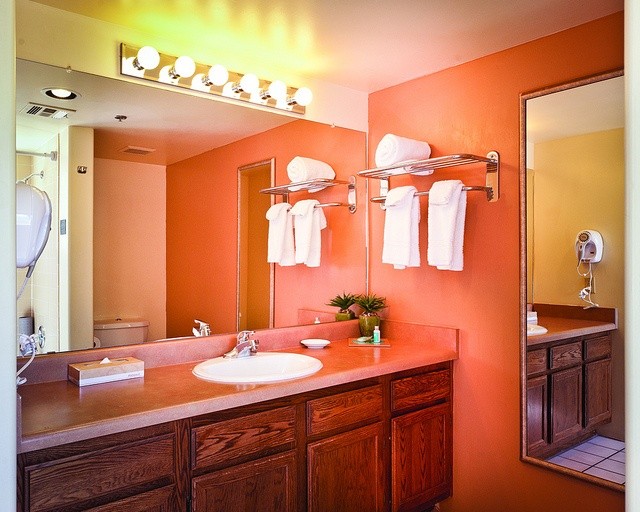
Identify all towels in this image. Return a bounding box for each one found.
[375,133,436,176]
[265,203,295,268]
[290,199,326,269]
[286,157,335,193]
[427,180,468,271]
[381,186,422,271]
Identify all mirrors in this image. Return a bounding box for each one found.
[518,68,625,491]
[17,59,367,357]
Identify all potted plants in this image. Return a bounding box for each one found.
[326,290,363,321]
[355,292,389,340]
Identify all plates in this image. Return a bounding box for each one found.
[301,339,331,349]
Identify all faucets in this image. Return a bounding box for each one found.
[235,326,261,353]
[192,319,210,337]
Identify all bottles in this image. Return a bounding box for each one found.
[314,318,320,323]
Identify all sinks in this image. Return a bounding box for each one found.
[193,350,323,385]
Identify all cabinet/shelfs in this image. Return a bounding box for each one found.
[306,374,386,510]
[583,330,613,430]
[523,343,548,454]
[391,359,454,511]
[551,339,582,442]
[188,392,307,511]
[34,418,188,510]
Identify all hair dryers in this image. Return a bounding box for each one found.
[578,231,590,265]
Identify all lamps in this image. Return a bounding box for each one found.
[268,81,286,101]
[170,57,196,79]
[208,65,228,88]
[294,88,312,107]
[239,72,259,96]
[132,45,161,72]
